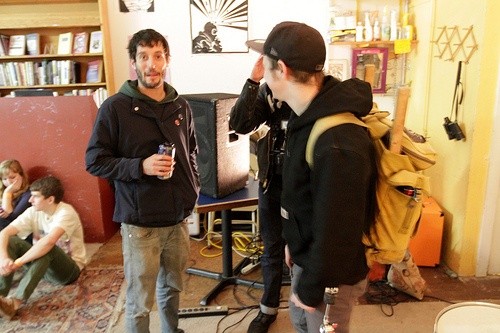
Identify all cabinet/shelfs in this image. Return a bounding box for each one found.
[408,196,444,267]
[0,0,114,110]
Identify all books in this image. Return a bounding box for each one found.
[0,31,103,97]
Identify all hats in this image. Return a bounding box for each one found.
[246,21,327,71]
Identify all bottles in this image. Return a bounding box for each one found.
[355,22,363,41]
[355,61,365,80]
[156,143,176,179]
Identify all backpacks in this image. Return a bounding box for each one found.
[305,103,437,264]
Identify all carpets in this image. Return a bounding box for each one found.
[0,263,128,333]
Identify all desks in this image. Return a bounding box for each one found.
[183,174,264,306]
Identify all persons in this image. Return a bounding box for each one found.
[245,21,376,333]
[228,56,292,333]
[0,160,32,231]
[0,175,86,320]
[85,30,201,333]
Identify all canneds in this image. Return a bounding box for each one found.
[157,142,175,180]
[402,189,414,197]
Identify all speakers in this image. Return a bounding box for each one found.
[179,92,249,199]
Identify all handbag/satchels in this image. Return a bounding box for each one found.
[387,251,428,300]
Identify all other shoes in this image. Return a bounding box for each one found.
[247,311,277,333]
[0,295,18,320]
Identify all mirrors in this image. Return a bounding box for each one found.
[352,47,388,94]
